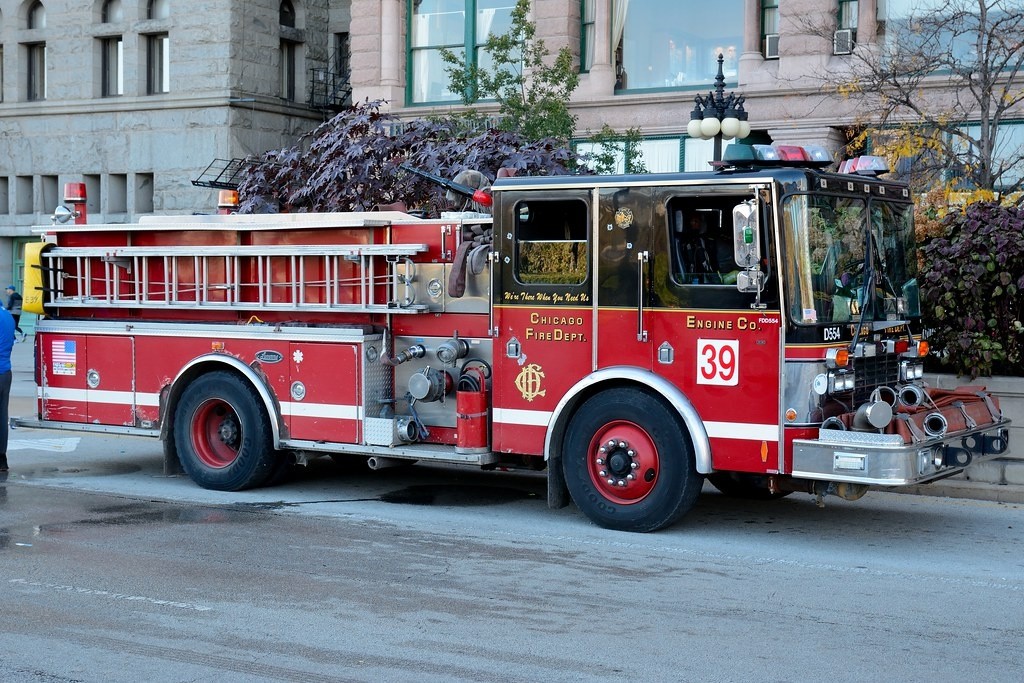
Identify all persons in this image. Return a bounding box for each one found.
[0,300,16,470]
[5,285,27,343]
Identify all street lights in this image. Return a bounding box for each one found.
[687,52,752,172]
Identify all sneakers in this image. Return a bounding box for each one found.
[14,339,19,344]
[21,332,27,342]
[0,463,9,471]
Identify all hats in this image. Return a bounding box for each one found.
[5,285,16,291]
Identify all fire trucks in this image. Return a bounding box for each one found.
[9,142,1015,534]
